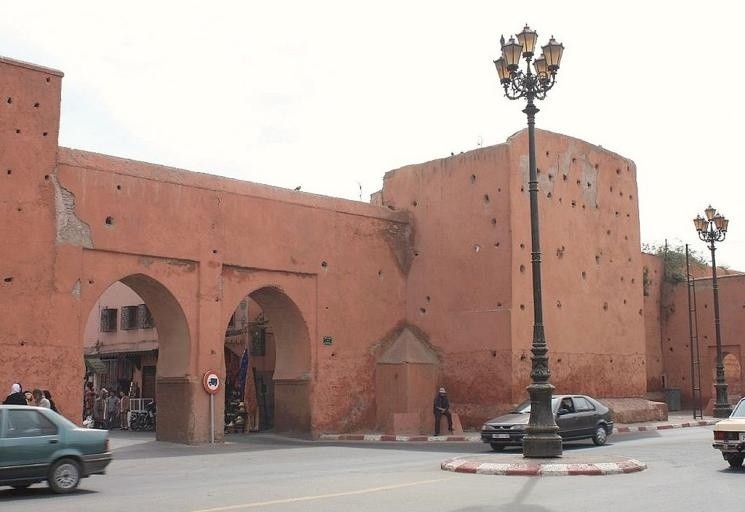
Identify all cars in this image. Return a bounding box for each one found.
[0,405,113,494]
[480,395,614,449]
[712,394,745,468]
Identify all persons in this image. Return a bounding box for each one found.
[434,388,456,436]
[2,382,59,413]
[82,385,130,431]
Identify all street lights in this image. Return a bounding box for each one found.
[694,202,730,419]
[495,20,565,458]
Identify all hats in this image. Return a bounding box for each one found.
[439,388,446,394]
